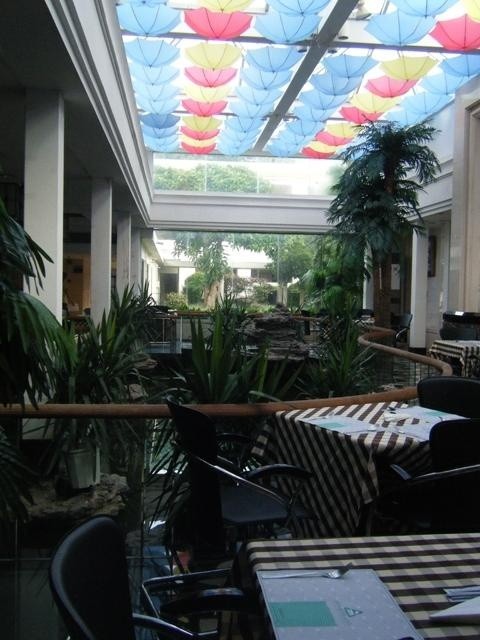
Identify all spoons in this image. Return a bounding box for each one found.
[343,423,382,435]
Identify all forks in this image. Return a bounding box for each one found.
[307,411,336,420]
[261,561,353,580]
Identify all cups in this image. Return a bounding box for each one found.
[407,397,420,410]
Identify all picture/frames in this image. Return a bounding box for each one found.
[428,236,436,278]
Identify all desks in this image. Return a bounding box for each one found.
[427,339,480,376]
[229,533,480,639]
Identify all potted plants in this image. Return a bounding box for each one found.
[44,280,169,493]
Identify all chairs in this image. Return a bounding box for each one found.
[389,312,412,346]
[45,514,246,640]
